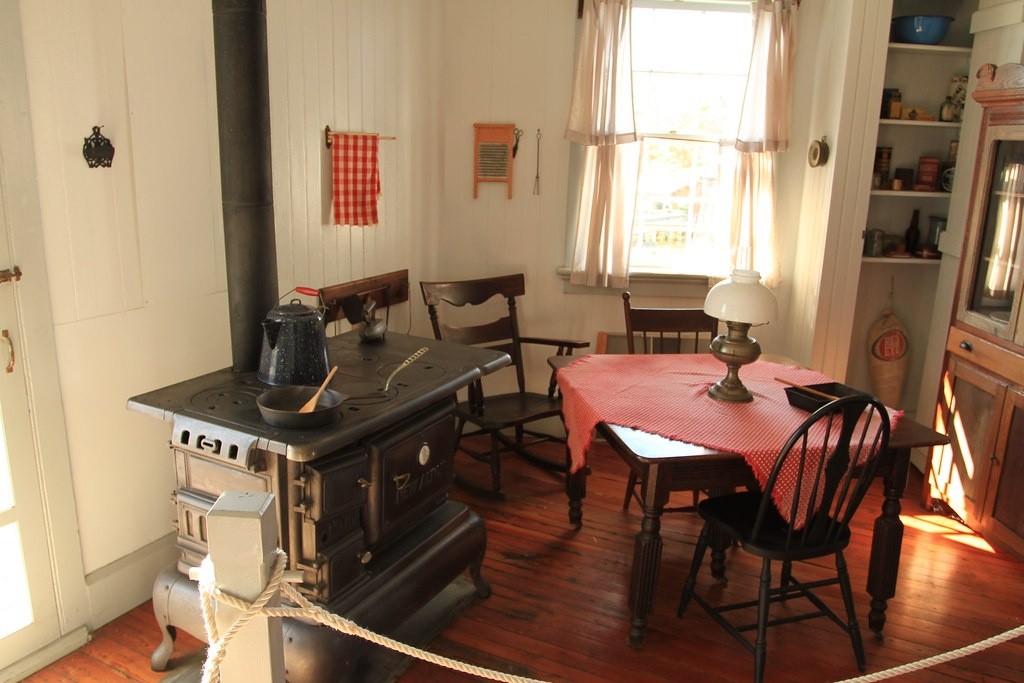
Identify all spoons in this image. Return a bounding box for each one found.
[298,366,338,412]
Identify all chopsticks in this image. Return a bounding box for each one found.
[774,376,847,400]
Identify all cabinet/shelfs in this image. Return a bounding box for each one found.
[923,89,1024,558]
[858,44,972,264]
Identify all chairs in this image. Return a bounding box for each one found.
[675,394,891,683]
[620,292,729,513]
[418,272,591,501]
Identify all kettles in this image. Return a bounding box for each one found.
[257,286,330,389]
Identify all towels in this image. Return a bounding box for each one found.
[332,132,383,228]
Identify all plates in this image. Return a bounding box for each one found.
[989,311,1011,323]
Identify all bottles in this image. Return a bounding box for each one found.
[939,96,956,122]
[904,208,921,255]
[938,140,958,192]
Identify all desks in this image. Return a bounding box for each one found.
[554,350,950,650]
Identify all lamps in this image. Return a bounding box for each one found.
[703,269,778,403]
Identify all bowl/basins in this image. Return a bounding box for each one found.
[255,386,350,430]
[892,15,955,45]
[783,382,867,415]
